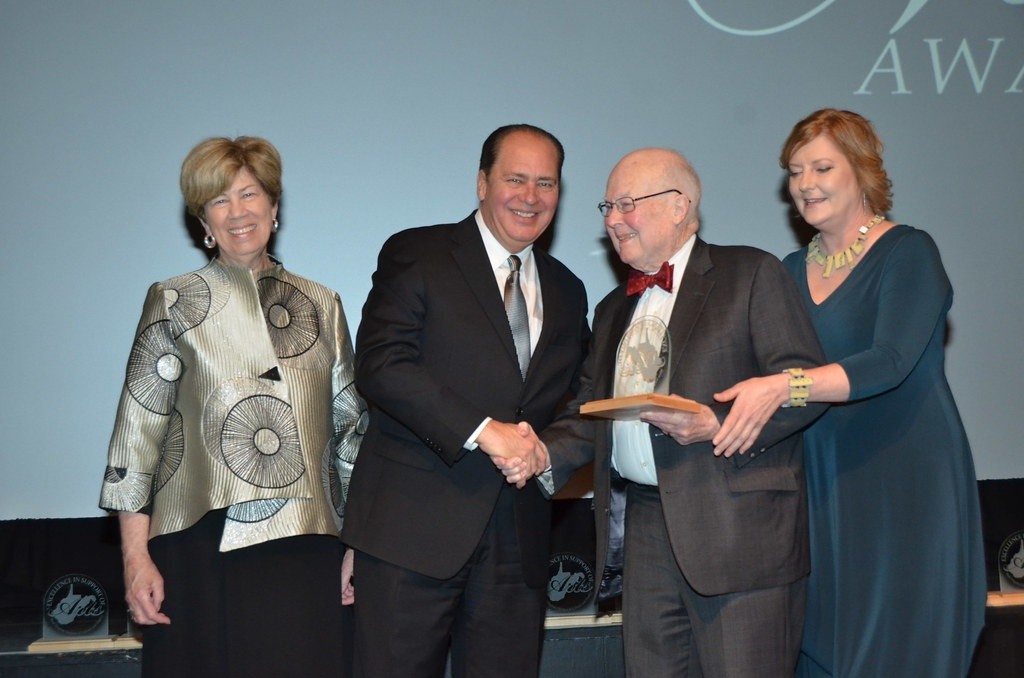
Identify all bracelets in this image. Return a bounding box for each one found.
[784,366,813,409]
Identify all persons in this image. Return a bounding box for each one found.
[98,136,368,678]
[343,125,591,678]
[489,107,987,678]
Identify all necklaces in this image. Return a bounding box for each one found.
[802,215,889,276]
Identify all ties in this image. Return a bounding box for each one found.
[504,255,531,382]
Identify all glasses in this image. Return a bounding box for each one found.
[598,190,691,217]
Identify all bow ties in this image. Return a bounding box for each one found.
[626,262,674,297]
